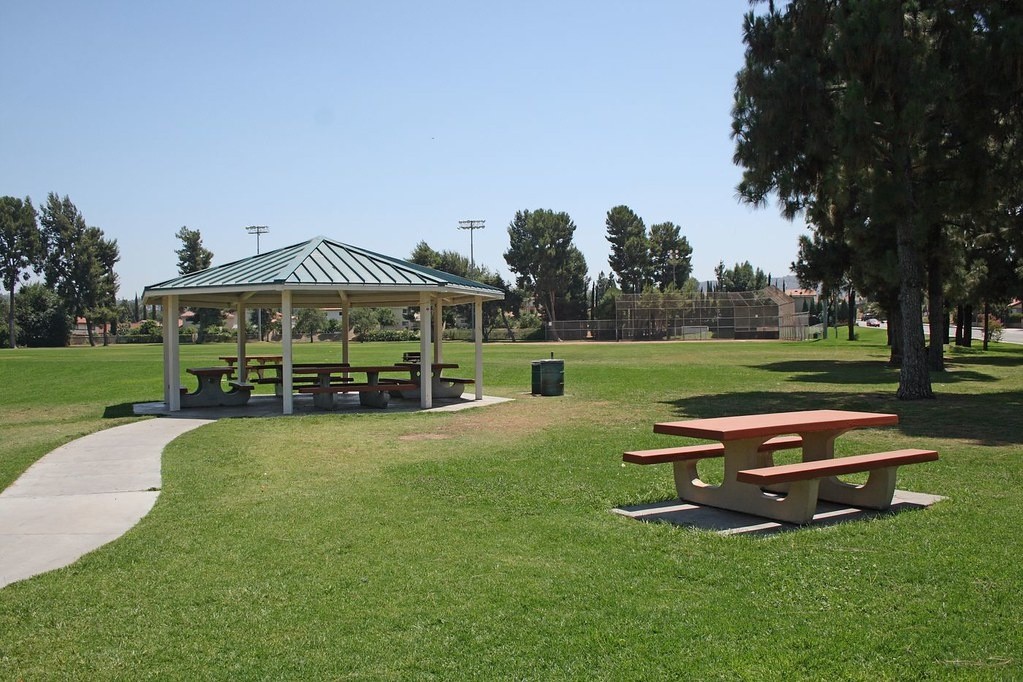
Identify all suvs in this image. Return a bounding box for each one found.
[861,313,872,321]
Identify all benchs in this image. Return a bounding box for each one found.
[622,437,802,504]
[737,449,940,524]
[173,375,476,409]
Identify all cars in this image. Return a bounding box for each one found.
[866,318,881,327]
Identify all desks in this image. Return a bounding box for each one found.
[293,366,410,409]
[184,368,234,406]
[249,361,349,398]
[219,356,282,380]
[653,409,900,523]
[395,359,459,399]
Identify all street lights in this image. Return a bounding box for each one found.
[669,249,679,340]
[246,225,270,341]
[457,219,486,341]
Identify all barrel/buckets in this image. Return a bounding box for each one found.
[532,360,541,394]
[541,358,565,396]
